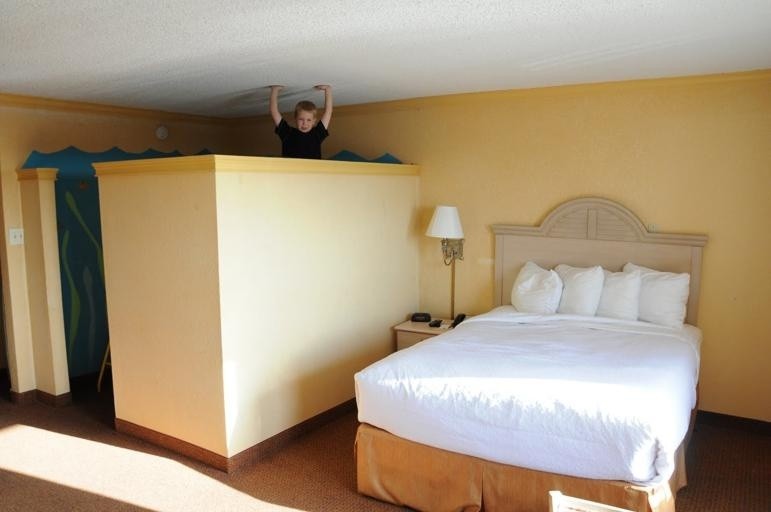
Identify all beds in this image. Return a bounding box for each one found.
[353,197,708,512]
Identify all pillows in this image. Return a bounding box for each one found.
[596,269,640,323]
[510,261,563,315]
[623,262,690,330]
[554,263,605,317]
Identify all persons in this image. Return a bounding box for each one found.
[269,84,333,159]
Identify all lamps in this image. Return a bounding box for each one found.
[424,205,464,326]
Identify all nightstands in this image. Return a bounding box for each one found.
[393,318,454,351]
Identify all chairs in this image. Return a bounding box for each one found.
[549,489,633,512]
